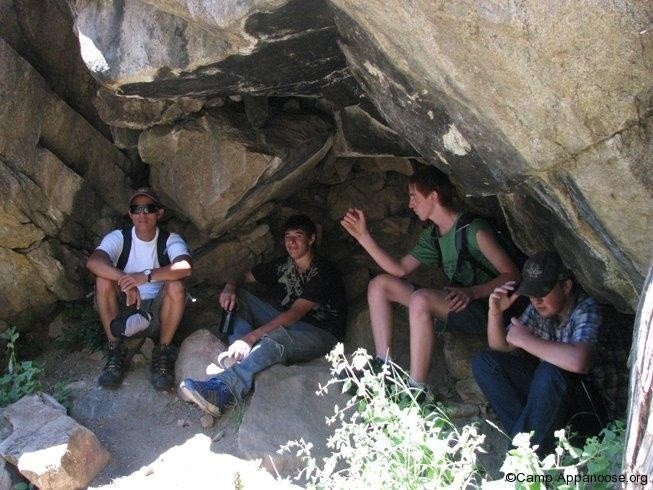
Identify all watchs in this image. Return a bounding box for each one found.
[142,267,152,282]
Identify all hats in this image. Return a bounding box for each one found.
[515,250,567,298]
[128,188,160,205]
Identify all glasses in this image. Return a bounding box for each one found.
[130,205,159,214]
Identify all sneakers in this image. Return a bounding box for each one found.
[217,351,240,370]
[151,343,177,391]
[180,377,231,418]
[341,359,427,405]
[99,340,130,388]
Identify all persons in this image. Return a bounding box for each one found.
[338,164,533,408]
[84,186,195,391]
[178,213,348,418]
[470,247,630,470]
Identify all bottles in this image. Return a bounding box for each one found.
[218,302,238,335]
